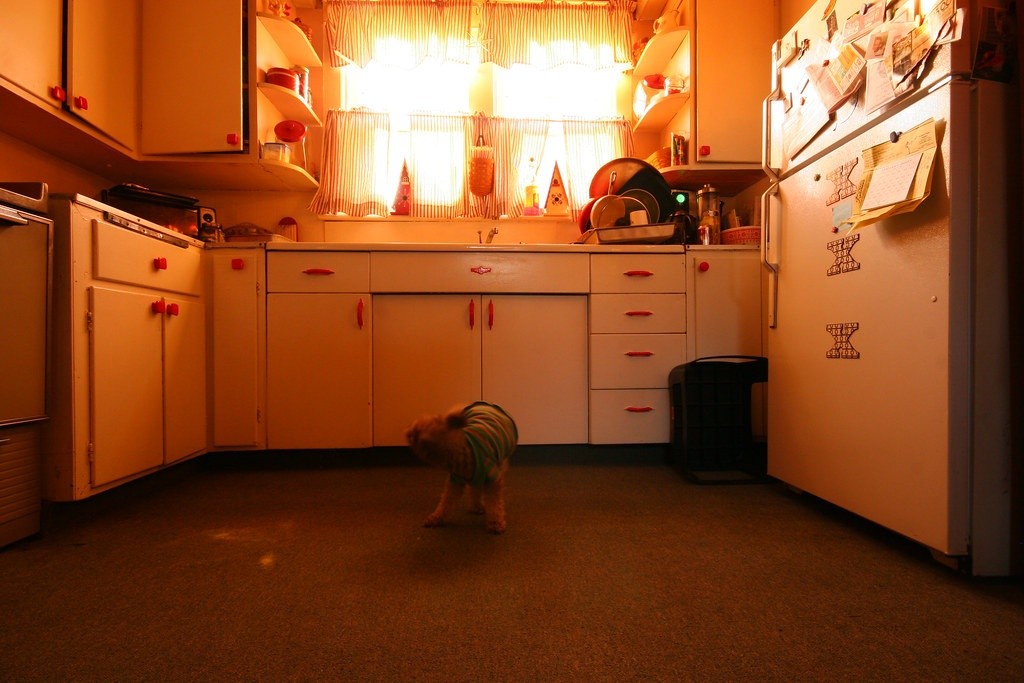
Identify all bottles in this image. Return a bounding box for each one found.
[696,183,721,245]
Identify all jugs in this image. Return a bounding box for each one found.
[652,9,680,35]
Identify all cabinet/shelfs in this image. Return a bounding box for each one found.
[0,0,783,547]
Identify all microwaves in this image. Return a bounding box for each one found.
[100,185,216,245]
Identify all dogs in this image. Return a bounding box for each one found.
[405,401,519,536]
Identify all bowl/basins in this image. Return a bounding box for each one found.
[722,226,763,247]
[577,189,659,235]
